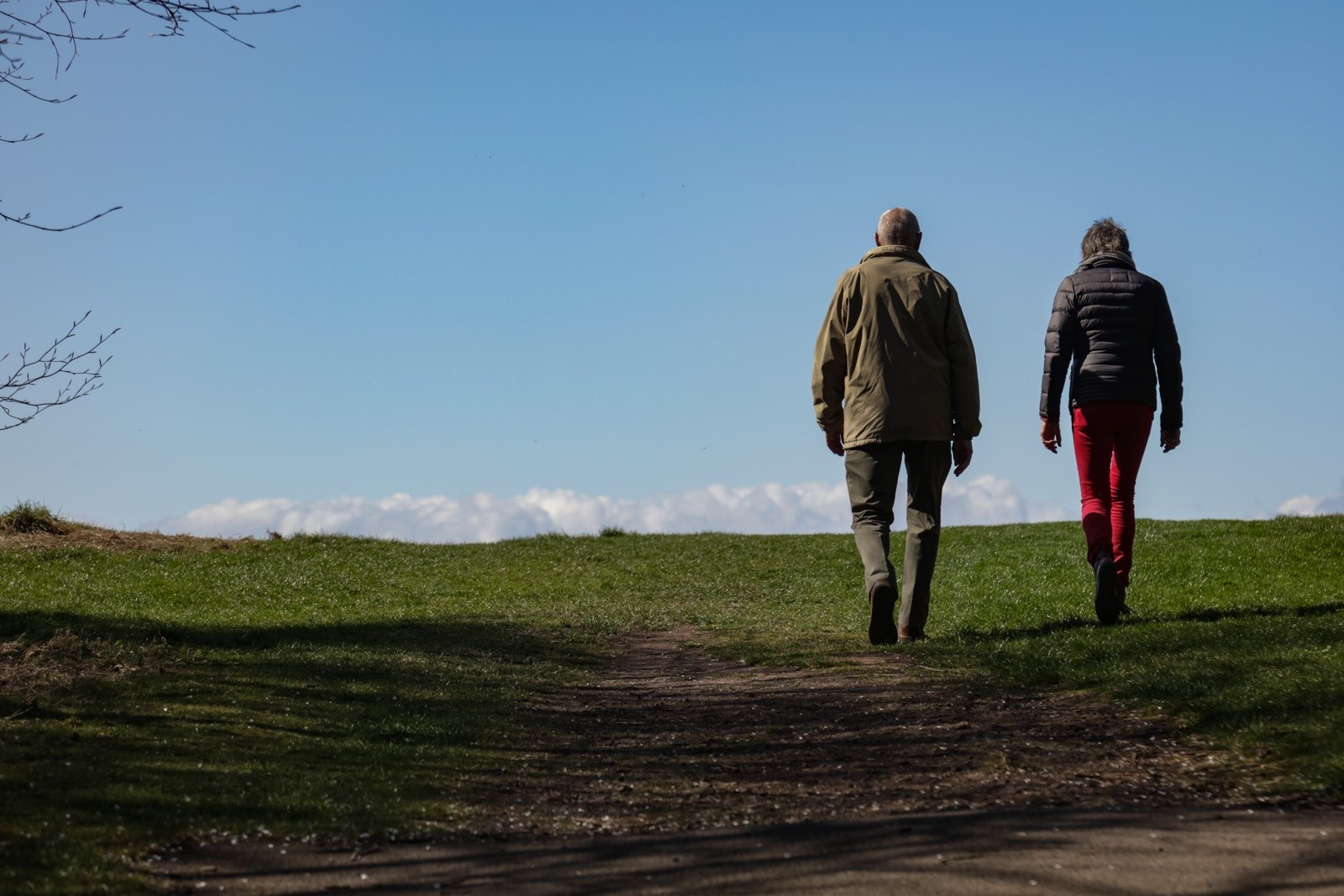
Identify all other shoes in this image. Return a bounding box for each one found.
[1095,558,1120,626]
[899,623,924,643]
[869,580,898,644]
[1119,603,1131,615]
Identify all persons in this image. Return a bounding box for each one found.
[1039,217,1184,626]
[812,207,982,645]
